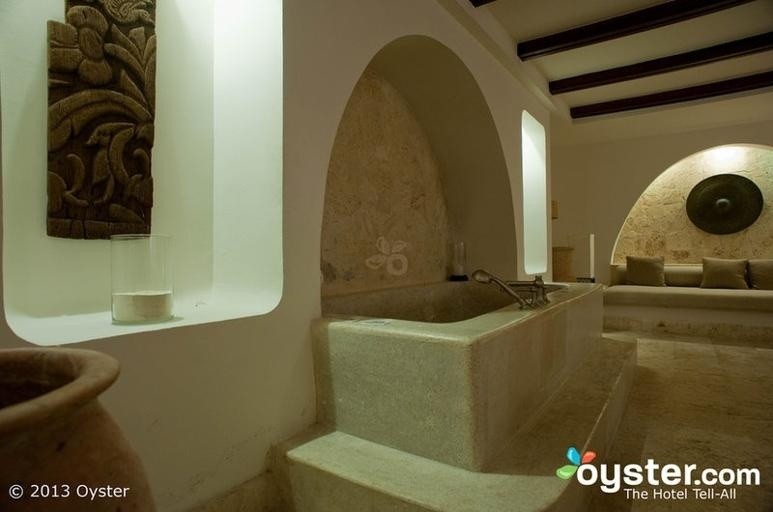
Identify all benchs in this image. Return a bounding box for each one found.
[603,265,773,340]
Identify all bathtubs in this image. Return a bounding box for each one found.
[311,276,604,471]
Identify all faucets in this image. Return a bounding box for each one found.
[472,267,534,313]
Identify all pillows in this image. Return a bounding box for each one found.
[748,259,773,289]
[699,257,749,289]
[626,255,666,287]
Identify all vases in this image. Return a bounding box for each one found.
[0,346,156,512]
